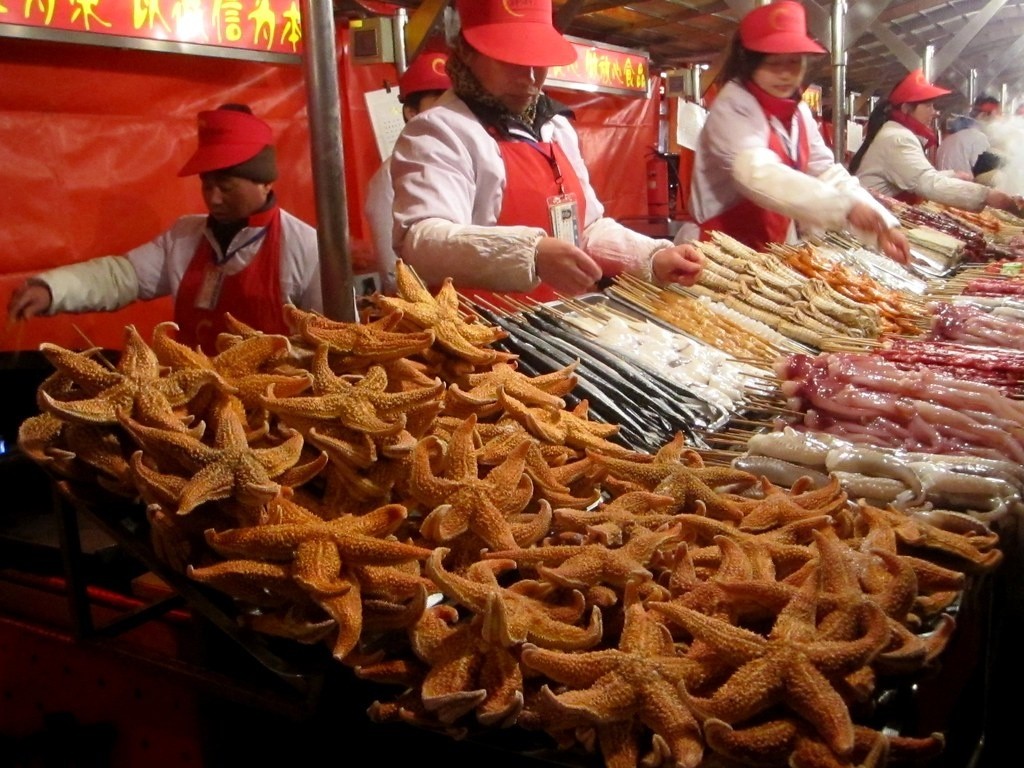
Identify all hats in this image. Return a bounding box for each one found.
[973,102,997,110]
[397,53,451,92]
[458,0,577,68]
[890,69,951,105]
[179,109,275,178]
[736,2,825,53]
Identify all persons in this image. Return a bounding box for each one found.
[562,210,571,218]
[363,52,1024,294]
[391,0,708,296]
[5,102,325,356]
[674,0,912,263]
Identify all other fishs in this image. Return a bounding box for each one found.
[472,192,1024,535]
